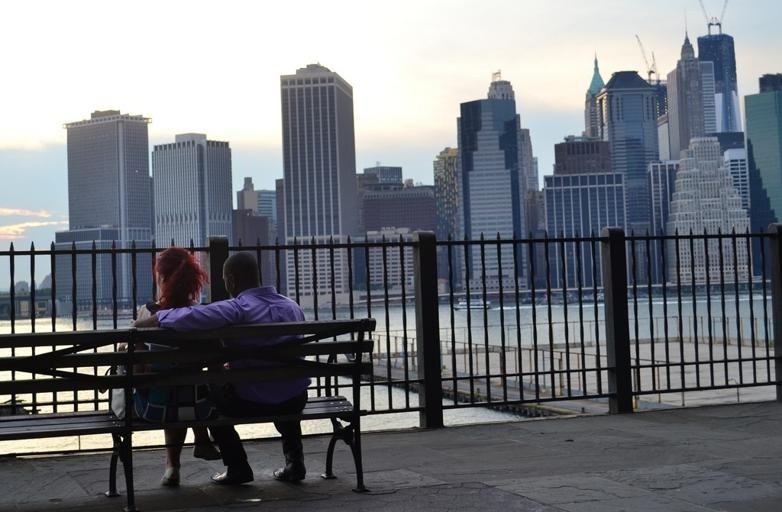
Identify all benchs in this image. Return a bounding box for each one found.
[0,318,377,512]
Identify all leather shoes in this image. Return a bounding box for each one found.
[210,470,255,485]
[273,463,306,481]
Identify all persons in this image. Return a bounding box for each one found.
[120,251,306,485]
[105,247,224,486]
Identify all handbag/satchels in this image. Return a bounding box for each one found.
[98,345,146,422]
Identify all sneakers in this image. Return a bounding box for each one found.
[193,445,223,461]
[161,467,180,486]
